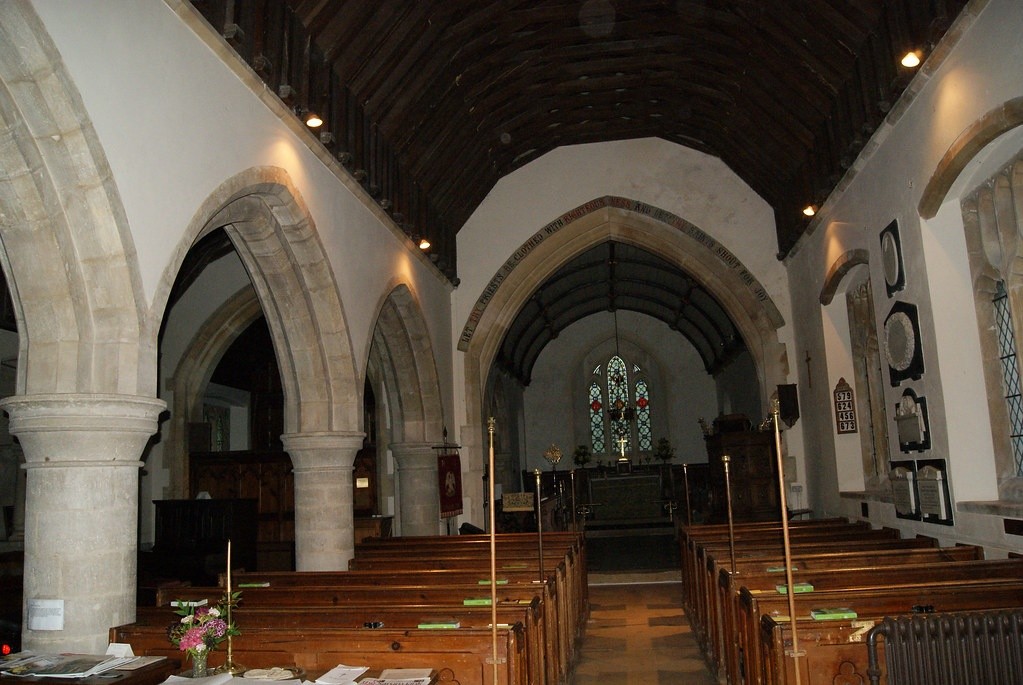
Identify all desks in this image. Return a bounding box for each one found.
[0,653,173,685]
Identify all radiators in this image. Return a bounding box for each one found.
[867,612,1023,685]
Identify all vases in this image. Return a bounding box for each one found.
[191,654,207,679]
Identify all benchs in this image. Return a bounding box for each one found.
[103,531,587,685]
[675,518,1023,683]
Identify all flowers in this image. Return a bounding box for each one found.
[171,588,244,660]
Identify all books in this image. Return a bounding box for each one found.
[418,621,460,628]
[464,596,499,605]
[171,598,208,606]
[157,673,301,685]
[0,653,167,678]
[479,578,508,584]
[315,663,370,685]
[489,623,512,626]
[238,581,270,587]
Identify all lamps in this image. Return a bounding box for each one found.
[417,238,430,251]
[802,203,818,216]
[302,111,323,127]
[901,49,924,68]
